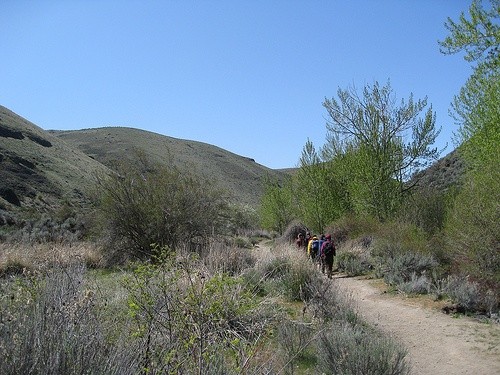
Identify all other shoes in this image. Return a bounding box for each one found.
[327,274,332,280]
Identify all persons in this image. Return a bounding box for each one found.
[322,233,336,279]
[295,231,326,273]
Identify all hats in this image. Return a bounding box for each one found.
[326,234,331,238]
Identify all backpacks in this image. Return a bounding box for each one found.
[325,241,333,256]
